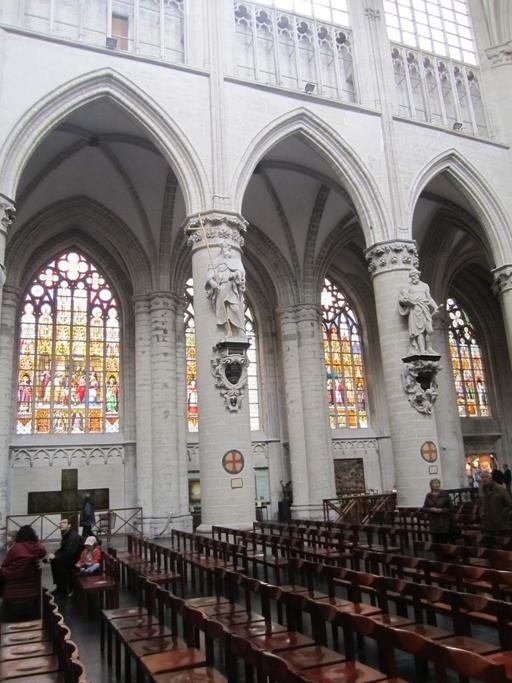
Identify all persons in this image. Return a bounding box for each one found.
[478,471,511,537]
[1,525,47,596]
[205,238,247,336]
[279,479,291,501]
[49,518,85,595]
[491,464,503,483]
[326,376,366,411]
[454,375,487,408]
[81,491,102,544]
[16,364,120,434]
[423,478,456,560]
[75,535,101,574]
[186,379,198,413]
[397,265,440,354]
[502,464,511,490]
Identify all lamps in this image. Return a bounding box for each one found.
[305,84,314,94]
[453,122,462,130]
[106,37,117,49]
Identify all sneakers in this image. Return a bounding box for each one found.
[80,567,90,575]
[51,588,77,596]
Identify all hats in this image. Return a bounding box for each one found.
[83,535,97,546]
